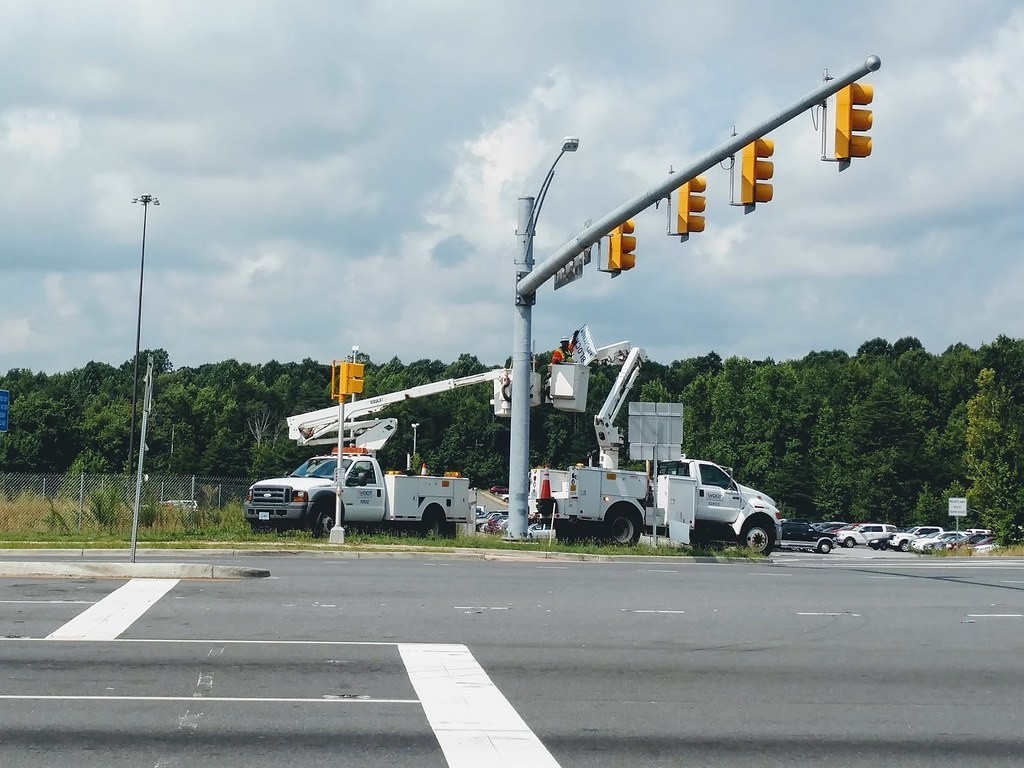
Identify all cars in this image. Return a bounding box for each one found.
[489,486,509,496]
[809,522,1002,554]
[472,493,557,543]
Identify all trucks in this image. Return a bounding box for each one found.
[242,362,513,540]
[525,322,784,560]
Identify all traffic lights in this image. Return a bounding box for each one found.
[740,138,775,203]
[677,175,707,233]
[337,359,365,397]
[607,219,637,270]
[833,81,874,161]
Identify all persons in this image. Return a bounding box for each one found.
[551,329,579,365]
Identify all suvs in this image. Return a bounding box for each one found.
[166,500,199,512]
[778,520,838,554]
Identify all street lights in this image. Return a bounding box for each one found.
[123,191,162,498]
[501,135,584,539]
[421,463,427,477]
[412,423,420,455]
